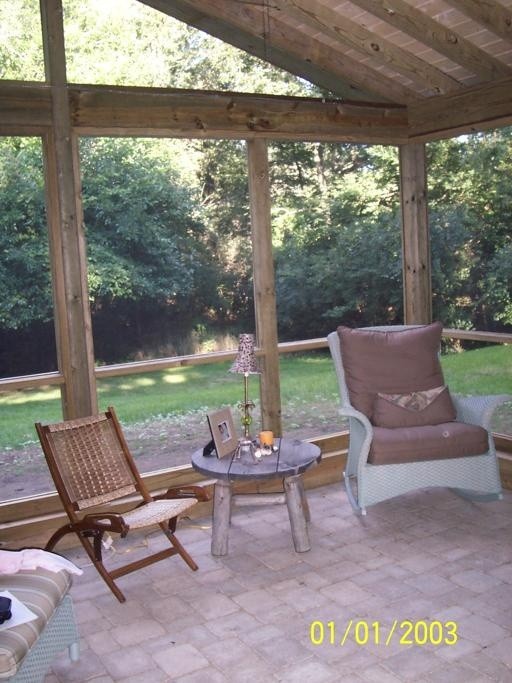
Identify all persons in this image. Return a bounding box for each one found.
[218,425,230,442]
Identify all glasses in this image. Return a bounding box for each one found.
[227,333,263,439]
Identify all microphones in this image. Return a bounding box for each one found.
[0,548,79,683]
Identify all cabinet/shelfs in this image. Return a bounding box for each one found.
[259,430,273,457]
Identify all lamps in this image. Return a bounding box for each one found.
[35,405,211,602]
[326,321,504,517]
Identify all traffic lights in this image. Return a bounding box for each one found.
[338,320,445,421]
[371,384,456,428]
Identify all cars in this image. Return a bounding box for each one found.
[235,437,247,459]
[240,442,258,466]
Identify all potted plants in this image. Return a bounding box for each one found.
[202,407,238,459]
